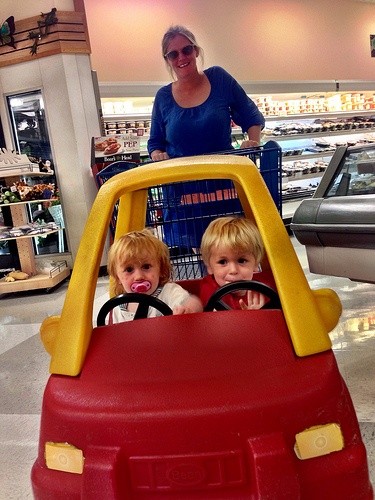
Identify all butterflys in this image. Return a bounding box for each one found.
[0,16,16,49]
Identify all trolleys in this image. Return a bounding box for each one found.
[92,141,284,355]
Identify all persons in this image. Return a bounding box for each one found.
[107,228,203,326]
[200,216,275,312]
[146,25,266,277]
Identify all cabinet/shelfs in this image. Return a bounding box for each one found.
[0,148,70,293]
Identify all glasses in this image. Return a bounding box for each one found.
[164,45,196,61]
